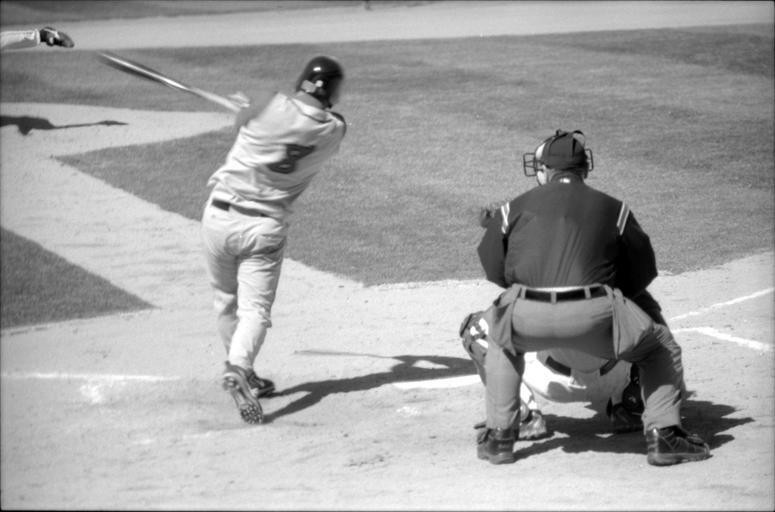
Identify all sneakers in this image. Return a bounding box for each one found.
[42,27,75,48]
[222,363,274,425]
[610,402,643,435]
[645,426,711,465]
[476,407,549,464]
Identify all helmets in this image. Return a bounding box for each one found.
[294,56,345,109]
[522,133,594,186]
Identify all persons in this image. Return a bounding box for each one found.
[478,129,710,465]
[460,289,667,439]
[201,56,346,425]
[0,27,74,50]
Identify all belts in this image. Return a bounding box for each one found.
[547,355,619,377]
[517,286,609,304]
[212,200,266,219]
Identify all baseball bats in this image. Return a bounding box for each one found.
[93,51,246,116]
